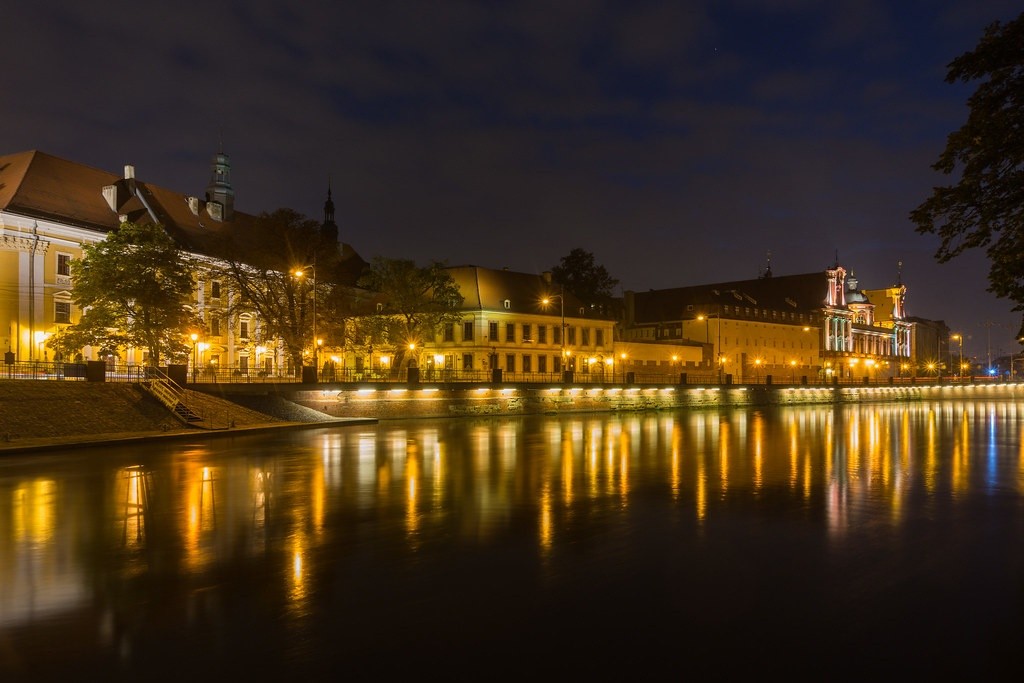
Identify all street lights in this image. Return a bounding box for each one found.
[929,364,934,386]
[952,335,962,385]
[804,327,825,386]
[409,343,415,359]
[698,313,721,384]
[673,355,678,382]
[191,333,198,384]
[904,364,908,377]
[963,364,968,377]
[543,295,566,381]
[722,359,726,383]
[565,351,571,371]
[295,264,316,366]
[1004,325,1018,380]
[977,322,1000,376]
[875,364,878,386]
[755,359,762,383]
[791,360,795,385]
[492,347,496,381]
[318,339,323,381]
[621,354,626,384]
